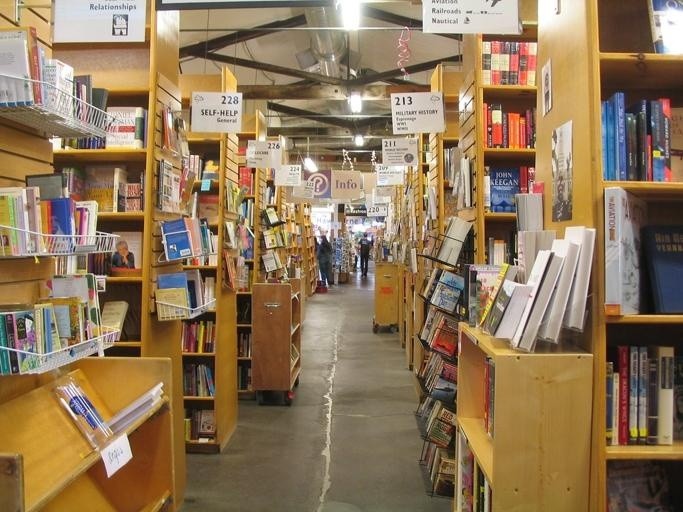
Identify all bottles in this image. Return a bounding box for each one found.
[53,370,115,451]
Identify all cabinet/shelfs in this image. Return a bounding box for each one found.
[1,1,187,511]
[236,110,319,402]
[373,0,681,512]
[330,236,354,276]
[176,70,238,455]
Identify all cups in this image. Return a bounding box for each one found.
[294,268,300,279]
[1,452,26,512]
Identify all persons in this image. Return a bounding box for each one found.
[352,230,383,278]
[314,236,334,284]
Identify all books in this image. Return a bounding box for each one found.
[388,0,683,512]
[0,26,320,440]
